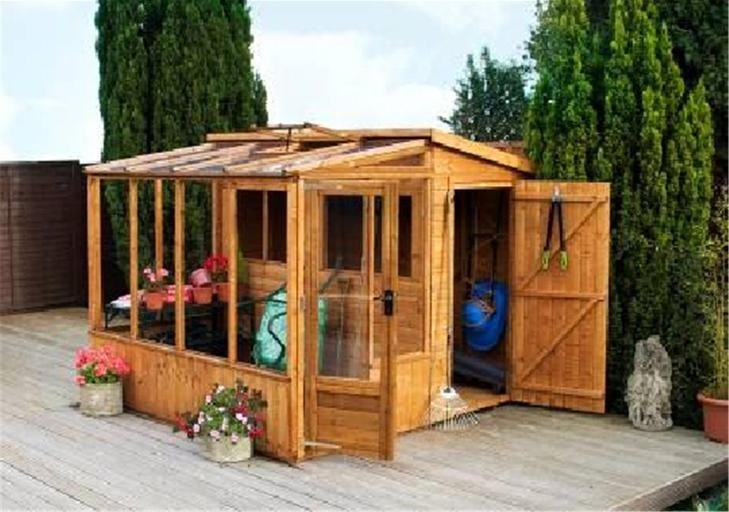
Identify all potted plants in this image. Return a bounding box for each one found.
[689,185,729,443]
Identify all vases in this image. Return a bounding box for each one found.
[191,268,212,286]
[81,383,123,417]
[191,285,213,305]
[146,292,163,309]
[208,436,252,463]
[216,282,229,302]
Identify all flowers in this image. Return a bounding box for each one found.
[75,343,131,385]
[140,267,169,291]
[174,380,267,443]
[204,253,228,282]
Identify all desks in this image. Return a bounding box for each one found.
[105,292,255,356]
